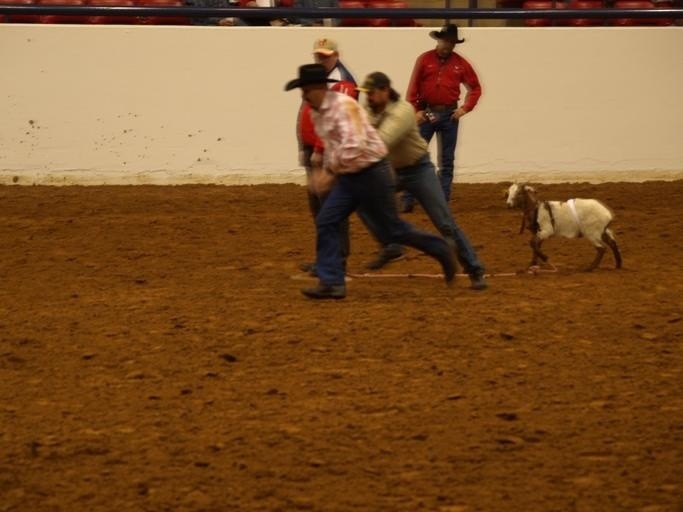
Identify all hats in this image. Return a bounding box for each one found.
[314,36,339,57]
[429,23,465,44]
[283,63,340,92]
[353,71,389,92]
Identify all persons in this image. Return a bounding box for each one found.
[353,71,487,290]
[1,1,682,26]
[399,24,481,214]
[296,37,360,279]
[284,63,459,299]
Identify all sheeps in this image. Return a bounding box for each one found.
[502,183,622,272]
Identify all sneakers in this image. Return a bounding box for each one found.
[441,244,457,281]
[300,262,317,274]
[367,252,404,270]
[301,284,345,298]
[468,268,486,289]
